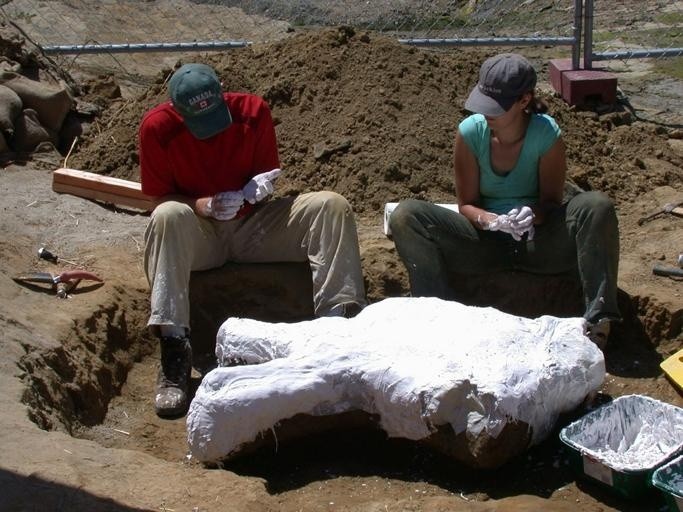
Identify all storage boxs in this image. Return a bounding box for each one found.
[558,393,683,499]
[651,455,683,512]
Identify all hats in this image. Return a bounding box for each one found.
[169,62,232,141]
[465,54,536,115]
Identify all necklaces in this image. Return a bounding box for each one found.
[491,128,527,147]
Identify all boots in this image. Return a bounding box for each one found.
[154,335,195,416]
[330,301,365,318]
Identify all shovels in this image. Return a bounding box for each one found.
[11,270,103,282]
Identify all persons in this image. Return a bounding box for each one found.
[137,62,369,418]
[388,51,629,366]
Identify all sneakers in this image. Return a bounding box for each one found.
[585,313,623,348]
